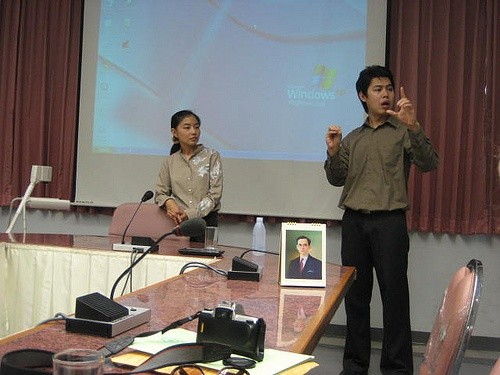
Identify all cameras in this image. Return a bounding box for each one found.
[197,300,267,362]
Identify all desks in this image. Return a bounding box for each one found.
[0,231,357,375]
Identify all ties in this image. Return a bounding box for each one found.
[299,258,305,272]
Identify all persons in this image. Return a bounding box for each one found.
[154,110,223,243]
[324,64,439,375]
[288,235,322,279]
[284,295,321,333]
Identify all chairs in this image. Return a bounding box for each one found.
[110,203,190,240]
[418,258,484,375]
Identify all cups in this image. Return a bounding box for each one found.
[205,226,219,251]
[52,349,105,375]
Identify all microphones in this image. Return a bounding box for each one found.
[121,190,154,244]
[110,217,206,300]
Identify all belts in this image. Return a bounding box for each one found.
[345,207,405,215]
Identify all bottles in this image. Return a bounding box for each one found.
[252,217,266,256]
